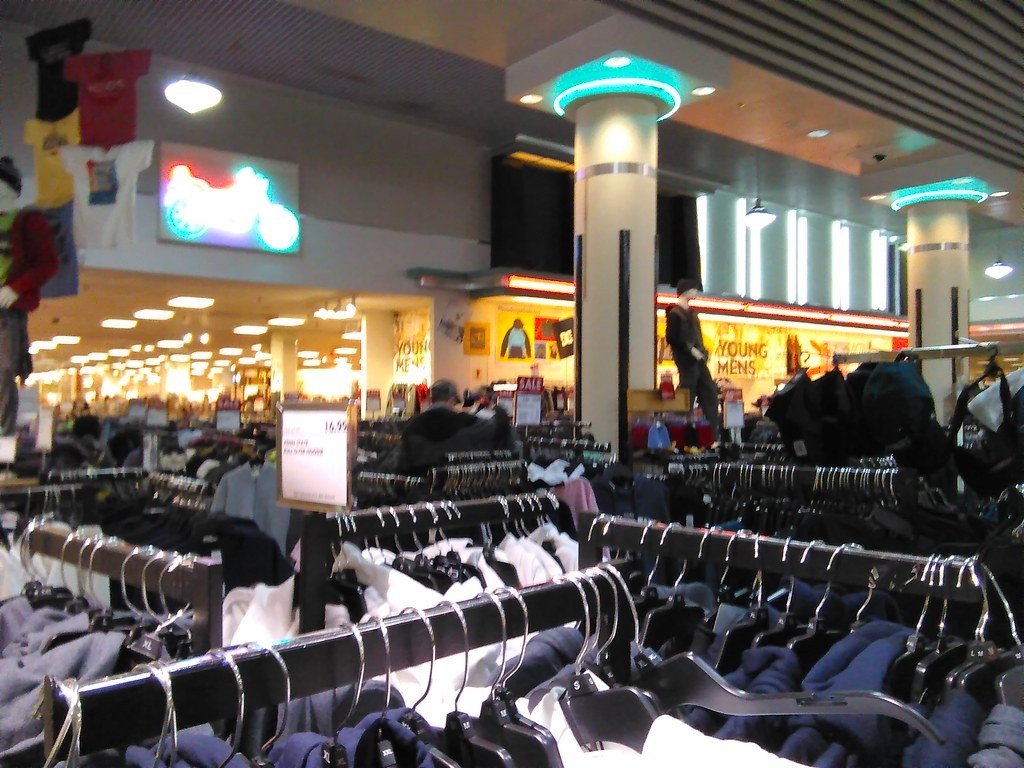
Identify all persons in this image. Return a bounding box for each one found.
[399,379,481,475]
[666,279,721,452]
[0,154,59,437]
[73,414,142,464]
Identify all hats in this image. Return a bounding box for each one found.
[0,155,21,192]
[778,356,945,466]
[431,382,461,405]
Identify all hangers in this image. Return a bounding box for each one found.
[1,339,1024,768]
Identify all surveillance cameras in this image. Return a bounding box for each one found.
[873,154,885,162]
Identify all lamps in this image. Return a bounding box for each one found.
[985,224,1013,280]
[164,56,224,115]
[742,155,778,229]
